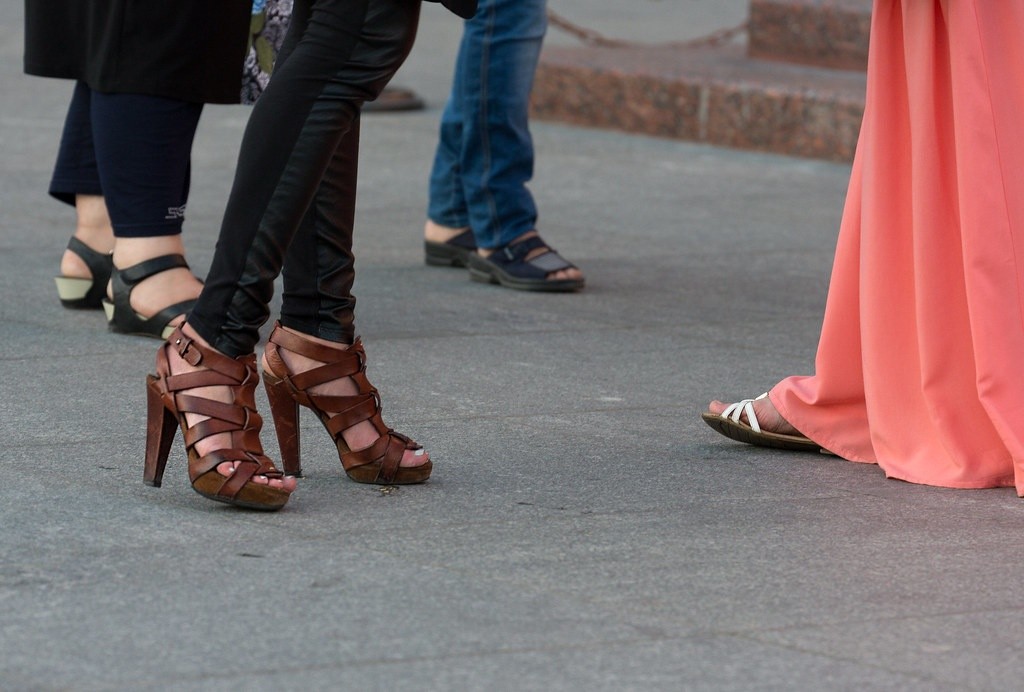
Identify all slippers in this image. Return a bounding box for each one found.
[422,231,475,266]
[467,228,585,292]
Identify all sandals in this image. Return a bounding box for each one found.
[58,235,207,342]
[702,391,846,450]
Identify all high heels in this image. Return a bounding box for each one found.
[261,321,432,486]
[142,317,292,511]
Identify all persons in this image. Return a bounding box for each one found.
[140,0,433,513]
[424,0,585,292]
[702,0,1023,498]
[24,0,255,339]
